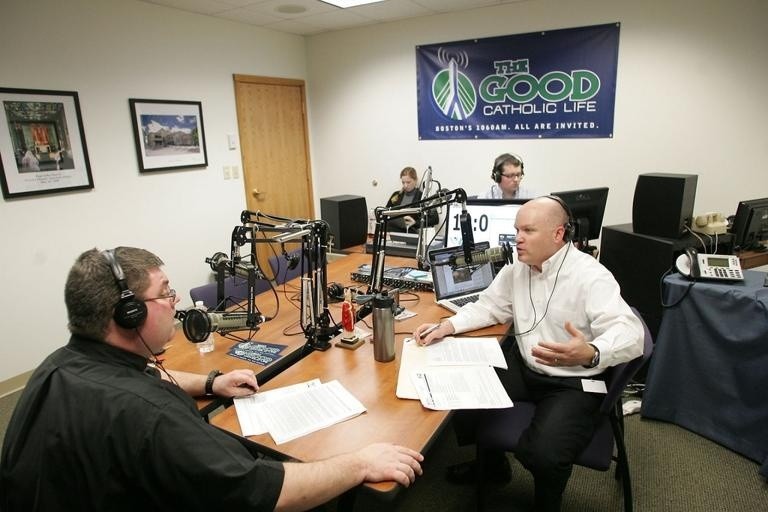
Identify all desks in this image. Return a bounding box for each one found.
[155,253,419,416]
[658,267,767,473]
[308,232,598,260]
[728,236,767,276]
[208,289,515,506]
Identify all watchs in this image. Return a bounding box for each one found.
[582,343,600,369]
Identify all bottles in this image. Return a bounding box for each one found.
[371,295,394,361]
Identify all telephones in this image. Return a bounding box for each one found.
[691,211,728,235]
[676,247,744,281]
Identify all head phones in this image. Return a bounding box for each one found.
[101,249,147,329]
[280,220,319,270]
[542,194,576,242]
[491,153,524,183]
[328,282,365,303]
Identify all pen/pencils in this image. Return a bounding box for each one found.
[407,323,440,343]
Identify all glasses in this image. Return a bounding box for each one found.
[142,289,176,302]
[501,172,522,179]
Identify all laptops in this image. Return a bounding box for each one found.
[429,241,496,314]
[383,217,407,233]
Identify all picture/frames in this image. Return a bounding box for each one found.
[127,97,208,174]
[0,87,95,199]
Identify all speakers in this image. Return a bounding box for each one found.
[320,194,368,250]
[633,172,698,239]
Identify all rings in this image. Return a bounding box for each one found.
[553,357,558,365]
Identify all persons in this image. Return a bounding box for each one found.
[1,247,425,511]
[414,196,644,511]
[477,154,539,199]
[385,167,439,232]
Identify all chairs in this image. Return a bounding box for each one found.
[471,307,655,512]
[188,272,271,314]
[268,246,320,283]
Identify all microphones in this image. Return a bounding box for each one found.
[422,188,451,216]
[182,307,265,342]
[433,242,513,271]
[206,251,264,280]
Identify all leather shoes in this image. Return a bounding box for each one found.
[448,460,512,485]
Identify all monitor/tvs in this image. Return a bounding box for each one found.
[730,198,768,253]
[551,188,609,255]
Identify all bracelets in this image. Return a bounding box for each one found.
[205,369,226,396]
